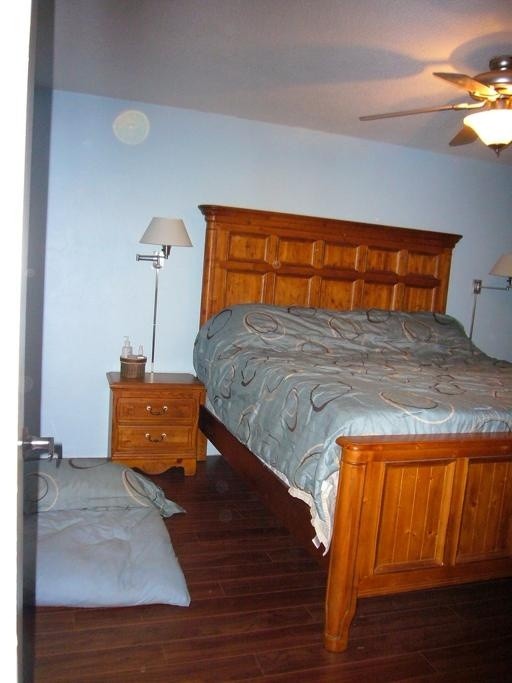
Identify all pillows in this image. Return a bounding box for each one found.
[38,455,186,519]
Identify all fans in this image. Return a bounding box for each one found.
[360,55,512,162]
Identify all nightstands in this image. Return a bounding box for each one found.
[108,374,209,480]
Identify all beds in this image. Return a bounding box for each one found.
[199,196,511,656]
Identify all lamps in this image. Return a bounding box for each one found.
[466,252,511,342]
[463,100,512,160]
[136,215,195,381]
